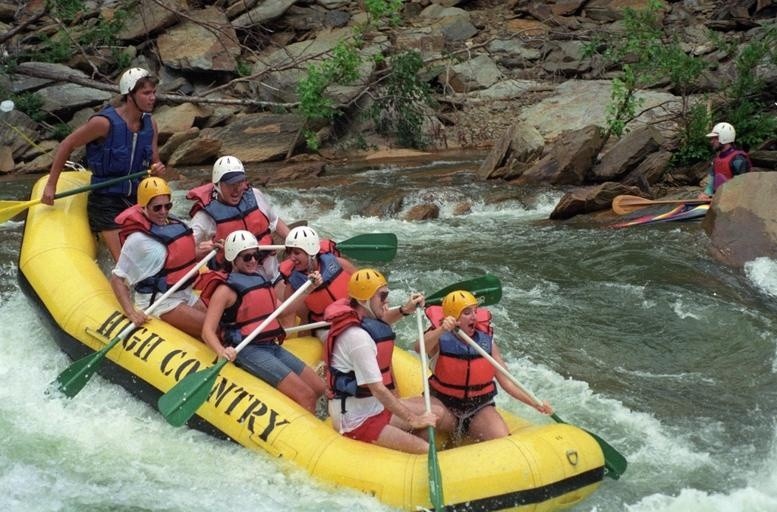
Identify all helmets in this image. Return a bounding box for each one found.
[223,230,259,262]
[136,177,171,207]
[347,269,387,301]
[284,226,320,256]
[211,155,245,183]
[119,67,151,95]
[441,290,478,320]
[704,122,736,145]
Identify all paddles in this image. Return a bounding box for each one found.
[612,195,713,216]
[271,220,308,244]
[0,164,157,224]
[284,274,501,334]
[453,325,627,480]
[158,277,315,427]
[416,302,445,512]
[257,234,397,262]
[54,245,220,399]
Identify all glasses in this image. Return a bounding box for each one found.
[152,203,172,213]
[378,291,389,302]
[238,252,259,262]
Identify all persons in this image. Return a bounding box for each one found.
[186,152,291,284]
[200,228,327,416]
[696,121,750,203]
[321,268,457,456]
[405,290,553,443]
[40,66,166,264]
[281,225,426,355]
[107,174,224,343]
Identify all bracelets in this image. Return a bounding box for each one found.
[398,304,411,316]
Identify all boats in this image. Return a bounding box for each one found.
[608,195,711,230]
[17,170,604,511]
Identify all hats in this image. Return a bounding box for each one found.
[221,172,246,185]
